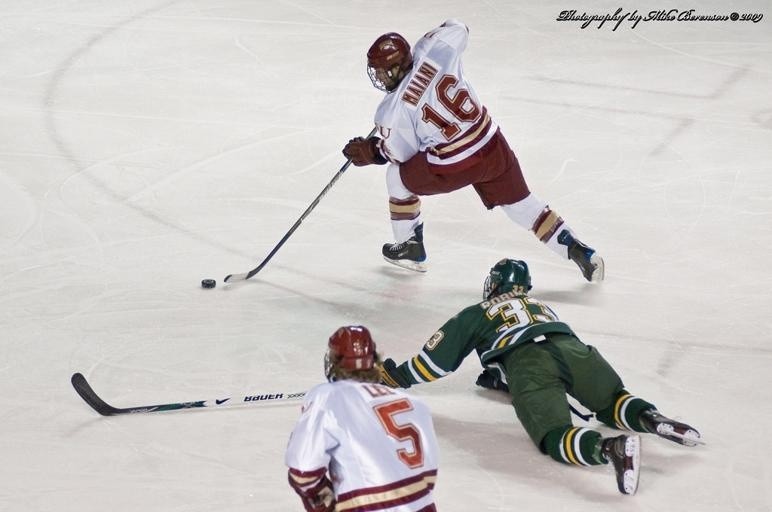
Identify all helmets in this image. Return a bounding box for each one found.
[367,33,413,94]
[324,326,376,382]
[483,259,531,302]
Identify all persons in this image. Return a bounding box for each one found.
[378,256,704,498]
[339,15,606,283]
[283,323,442,512]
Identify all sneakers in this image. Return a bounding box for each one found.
[601,434,627,493]
[380,243,426,266]
[569,241,597,283]
[638,409,701,447]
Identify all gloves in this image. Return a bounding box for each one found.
[343,137,388,166]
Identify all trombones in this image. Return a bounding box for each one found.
[223,129,377,281]
